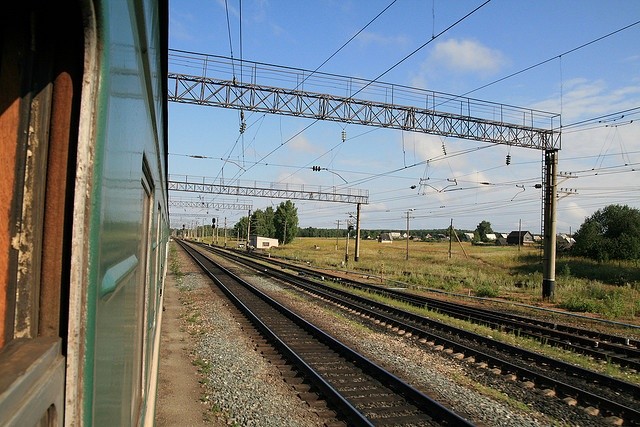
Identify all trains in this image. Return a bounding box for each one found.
[0,0,171,427]
